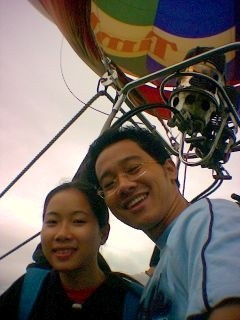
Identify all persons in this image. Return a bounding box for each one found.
[83,125,239,320]
[0,181,146,320]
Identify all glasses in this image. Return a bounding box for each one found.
[96,162,160,199]
